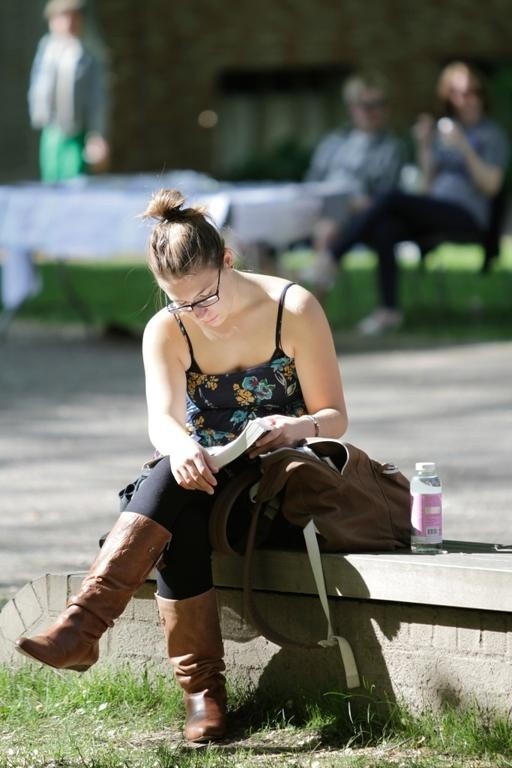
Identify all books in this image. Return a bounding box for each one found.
[205,418,274,470]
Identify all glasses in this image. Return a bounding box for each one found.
[165,239,225,314]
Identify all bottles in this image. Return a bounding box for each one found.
[408,462,443,554]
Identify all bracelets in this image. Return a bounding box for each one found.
[301,414,321,436]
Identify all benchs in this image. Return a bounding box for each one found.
[300,164,511,279]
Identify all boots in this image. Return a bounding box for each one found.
[14,511,173,672]
[154,585,227,743]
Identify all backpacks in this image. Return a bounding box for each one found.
[218,436,410,652]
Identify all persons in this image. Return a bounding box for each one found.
[327,60,512,338]
[25,0,114,183]
[236,68,412,308]
[14,188,349,743]
